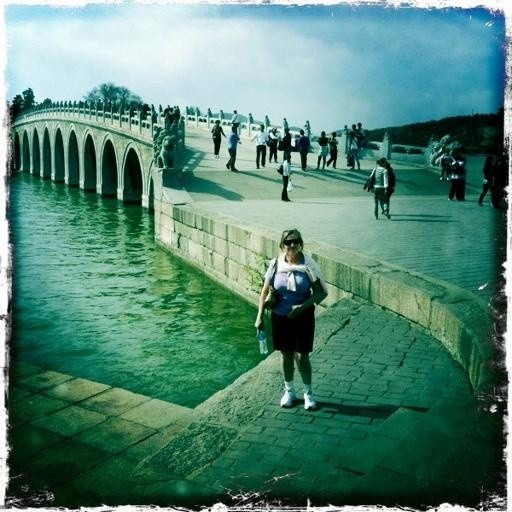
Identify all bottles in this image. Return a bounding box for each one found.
[257,328,268,354]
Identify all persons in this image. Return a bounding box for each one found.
[439,143,504,210]
[209,110,365,202]
[165,104,180,126]
[254,229,327,409]
[368,158,395,219]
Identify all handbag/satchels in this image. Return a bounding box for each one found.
[363,174,376,192]
[276,164,283,176]
[264,286,277,309]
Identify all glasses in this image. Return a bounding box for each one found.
[282,238,301,246]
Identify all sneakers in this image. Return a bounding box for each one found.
[280,389,297,407]
[384,213,391,220]
[303,392,316,410]
[225,163,239,172]
